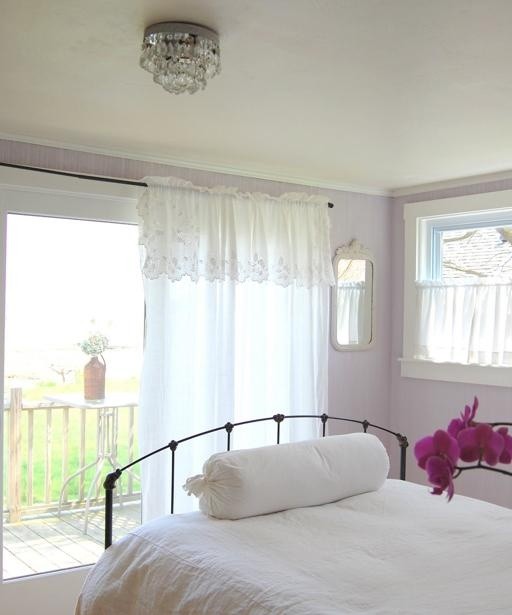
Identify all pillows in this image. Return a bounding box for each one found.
[183,432,393,521]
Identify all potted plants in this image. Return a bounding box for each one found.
[73,334,110,400]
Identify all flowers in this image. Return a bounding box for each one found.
[413,396,512,504]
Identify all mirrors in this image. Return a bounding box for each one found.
[328,237,377,352]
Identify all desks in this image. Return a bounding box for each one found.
[42,389,140,536]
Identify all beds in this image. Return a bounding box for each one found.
[72,414,511,614]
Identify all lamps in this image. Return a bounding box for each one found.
[139,21,222,97]
